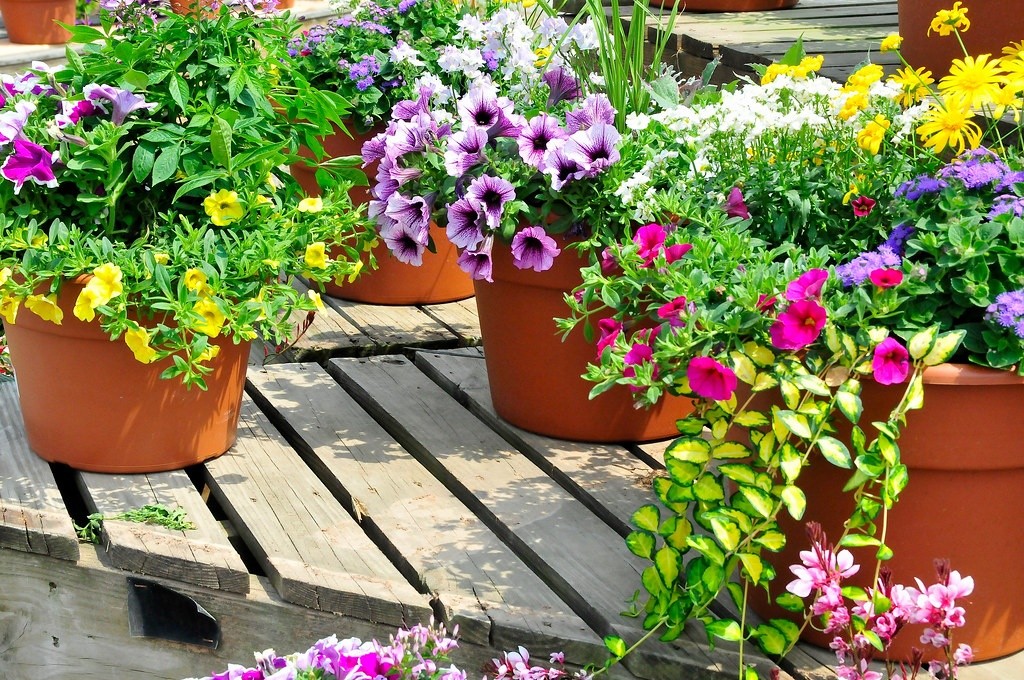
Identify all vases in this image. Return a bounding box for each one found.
[0,0,77,45]
[263,0,295,9]
[728,358,1024,668]
[170,0,222,19]
[289,110,475,307]
[471,207,712,443]
[1,261,253,477]
[898,0,1023,81]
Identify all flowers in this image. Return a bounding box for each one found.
[0,0,1024,680]
